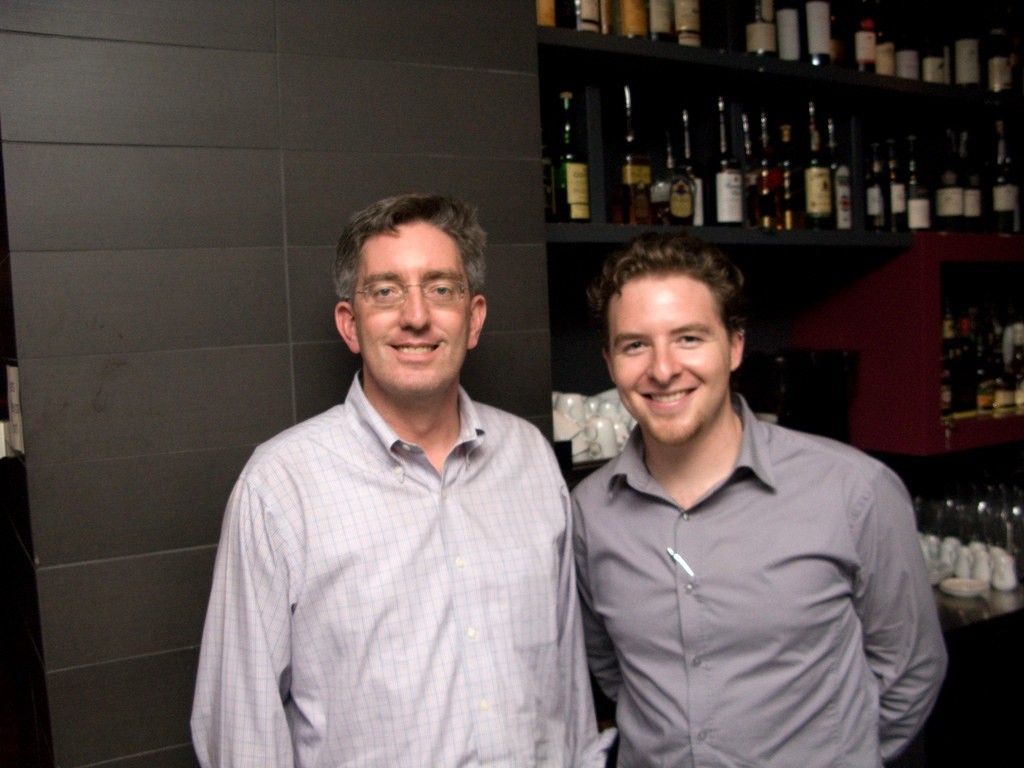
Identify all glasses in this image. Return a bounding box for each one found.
[350,280,470,307]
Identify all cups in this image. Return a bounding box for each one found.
[910,477,1024,599]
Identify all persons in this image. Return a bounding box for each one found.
[571,233,947,768]
[191,190,619,768]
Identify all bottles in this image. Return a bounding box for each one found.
[533,0,1024,421]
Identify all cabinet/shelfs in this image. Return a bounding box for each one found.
[539,25,1024,455]
